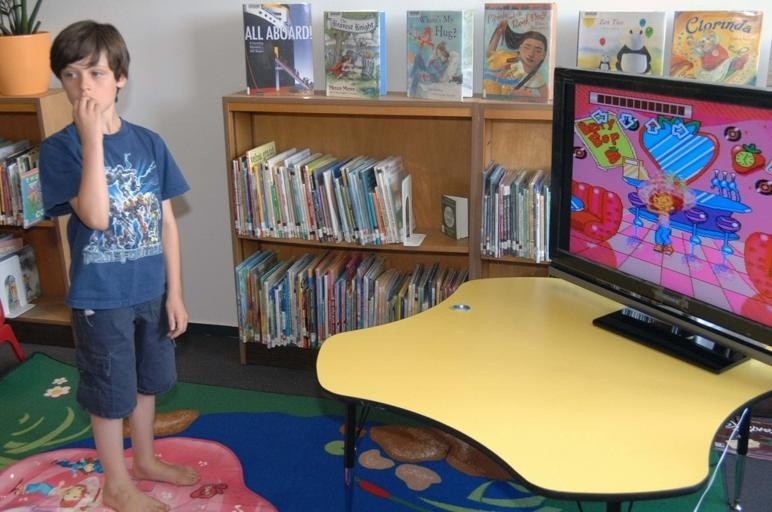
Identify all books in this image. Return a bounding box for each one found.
[244,2,556,101]
[480,160,551,263]
[440,194,468,240]
[0,138,61,318]
[234,250,469,348]
[576,10,763,86]
[233,140,416,246]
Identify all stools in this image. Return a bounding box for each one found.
[2,304,25,365]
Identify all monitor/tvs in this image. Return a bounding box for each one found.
[549,65,772,375]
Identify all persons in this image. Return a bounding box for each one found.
[639,181,689,256]
[38,21,199,512]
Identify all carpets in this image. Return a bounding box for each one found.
[0,348,735,512]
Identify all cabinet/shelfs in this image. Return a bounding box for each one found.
[221,85,554,371]
[0,88,80,348]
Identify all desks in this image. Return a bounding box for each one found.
[312,274,772,511]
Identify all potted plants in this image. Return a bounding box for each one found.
[1,0,53,102]
